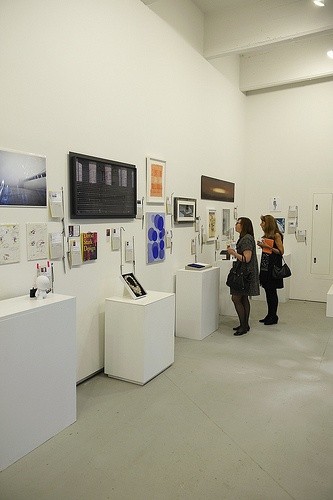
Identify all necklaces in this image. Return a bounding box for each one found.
[125,275,143,295]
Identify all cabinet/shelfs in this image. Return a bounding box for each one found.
[206,209,217,241]
[215,258,238,315]
[0,293,77,473]
[251,247,292,304]
[174,267,220,340]
[105,290,175,386]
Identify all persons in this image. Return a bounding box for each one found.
[226,218,260,335]
[258,215,284,325]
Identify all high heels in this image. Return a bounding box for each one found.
[259,314,278,325]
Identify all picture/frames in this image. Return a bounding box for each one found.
[146,156,166,204]
[173,197,197,222]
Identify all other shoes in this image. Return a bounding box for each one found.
[233,325,250,336]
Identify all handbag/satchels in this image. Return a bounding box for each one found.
[226,255,250,292]
[272,249,291,279]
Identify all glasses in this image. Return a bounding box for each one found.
[237,222,241,225]
